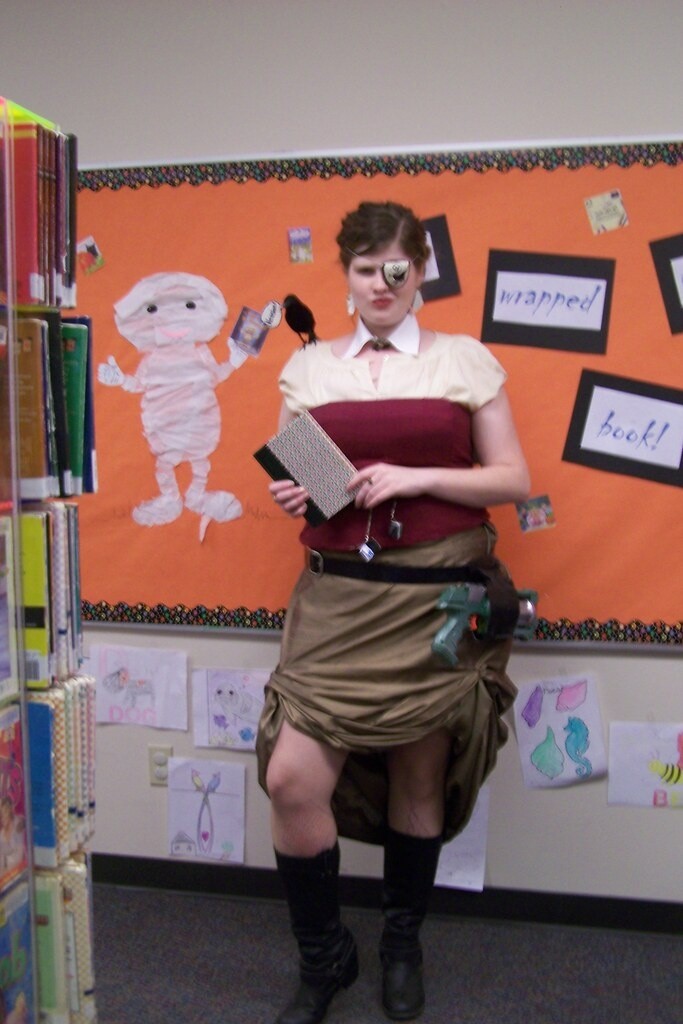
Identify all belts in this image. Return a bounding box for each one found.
[303,549,487,582]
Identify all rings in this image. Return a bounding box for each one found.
[366,476,374,485]
[273,495,278,503]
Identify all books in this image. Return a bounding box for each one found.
[1,120,96,1024]
[254,409,366,526]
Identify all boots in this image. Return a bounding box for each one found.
[379,827,442,1018]
[272,839,358,1024]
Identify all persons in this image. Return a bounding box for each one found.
[257,200,528,1024]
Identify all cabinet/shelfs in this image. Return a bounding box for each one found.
[0,97,98,1024]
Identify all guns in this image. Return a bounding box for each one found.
[431,584,540,669]
